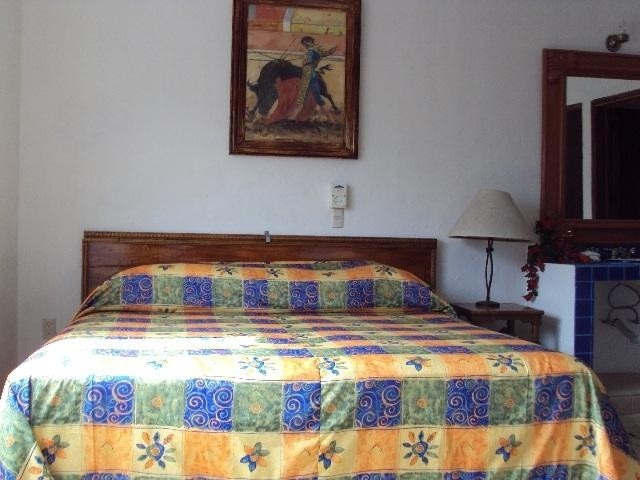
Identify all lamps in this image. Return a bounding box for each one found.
[448,189,534,307]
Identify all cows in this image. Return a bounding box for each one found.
[246,58,342,123]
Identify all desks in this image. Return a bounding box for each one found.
[452,302,544,344]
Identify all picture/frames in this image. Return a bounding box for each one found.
[229,0,362,160]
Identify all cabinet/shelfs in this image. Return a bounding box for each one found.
[529,260,640,373]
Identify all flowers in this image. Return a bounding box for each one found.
[521,209,591,300]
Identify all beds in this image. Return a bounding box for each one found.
[1,230,639,479]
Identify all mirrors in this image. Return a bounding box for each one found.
[541,48,640,246]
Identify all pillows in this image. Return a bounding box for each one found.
[81,259,452,315]
